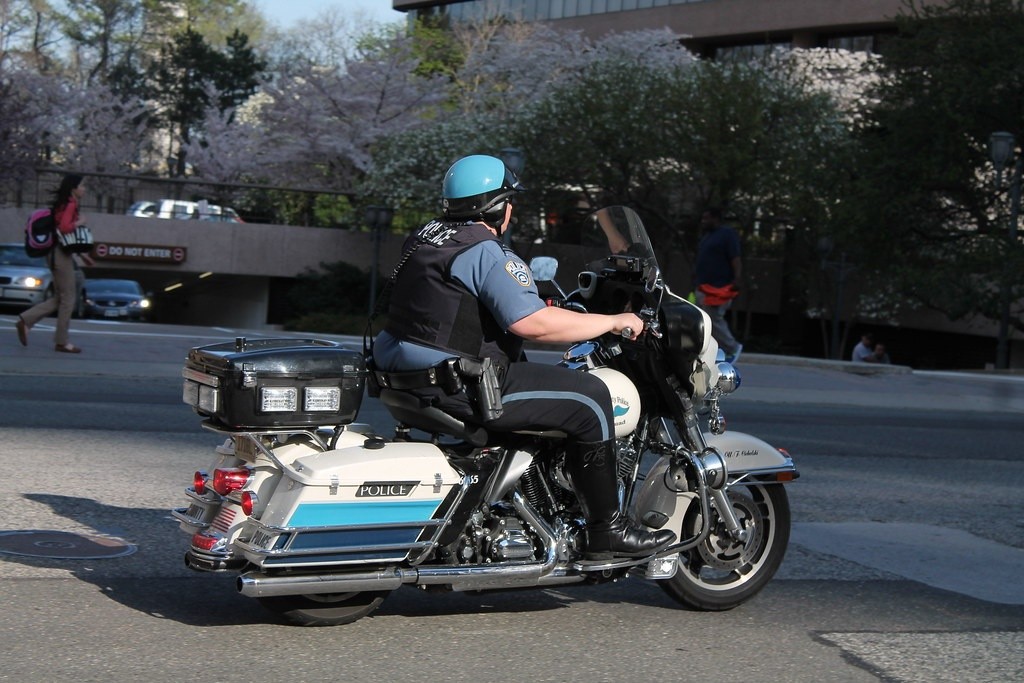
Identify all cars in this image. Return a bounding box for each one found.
[126,201,157,217]
[0,242,85,319]
[84,279,151,323]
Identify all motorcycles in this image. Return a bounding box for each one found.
[170,206,806,628]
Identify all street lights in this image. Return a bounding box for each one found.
[365,205,393,322]
[989,131,1024,368]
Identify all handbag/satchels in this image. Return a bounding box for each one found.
[696,283,742,308]
[53,226,95,255]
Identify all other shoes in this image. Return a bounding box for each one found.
[55,343,83,353]
[14,320,30,347]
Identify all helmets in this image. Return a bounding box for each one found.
[441,154,528,219]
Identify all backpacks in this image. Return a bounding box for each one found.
[23,208,55,258]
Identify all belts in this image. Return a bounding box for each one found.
[371,368,458,389]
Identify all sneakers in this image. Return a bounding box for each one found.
[722,343,743,367]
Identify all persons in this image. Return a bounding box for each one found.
[16,173,94,353]
[693,207,743,365]
[372,154,678,561]
[852,332,890,364]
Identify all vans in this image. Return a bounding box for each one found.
[154,198,244,223]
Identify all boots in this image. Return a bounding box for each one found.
[571,437,677,566]
